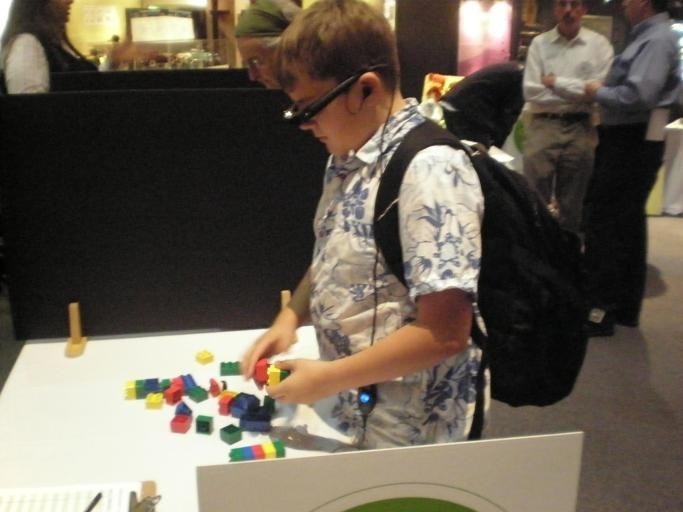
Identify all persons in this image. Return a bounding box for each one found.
[0,0,97,94]
[243,2,483,450]
[522,0,617,237]
[440,59,524,152]
[582,0,682,338]
[234,0,302,88]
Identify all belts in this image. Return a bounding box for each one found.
[535,110,590,122]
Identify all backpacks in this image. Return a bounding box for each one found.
[373,119,599,407]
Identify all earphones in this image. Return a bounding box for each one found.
[361,84,372,98]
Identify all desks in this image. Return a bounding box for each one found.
[2,323,361,512]
